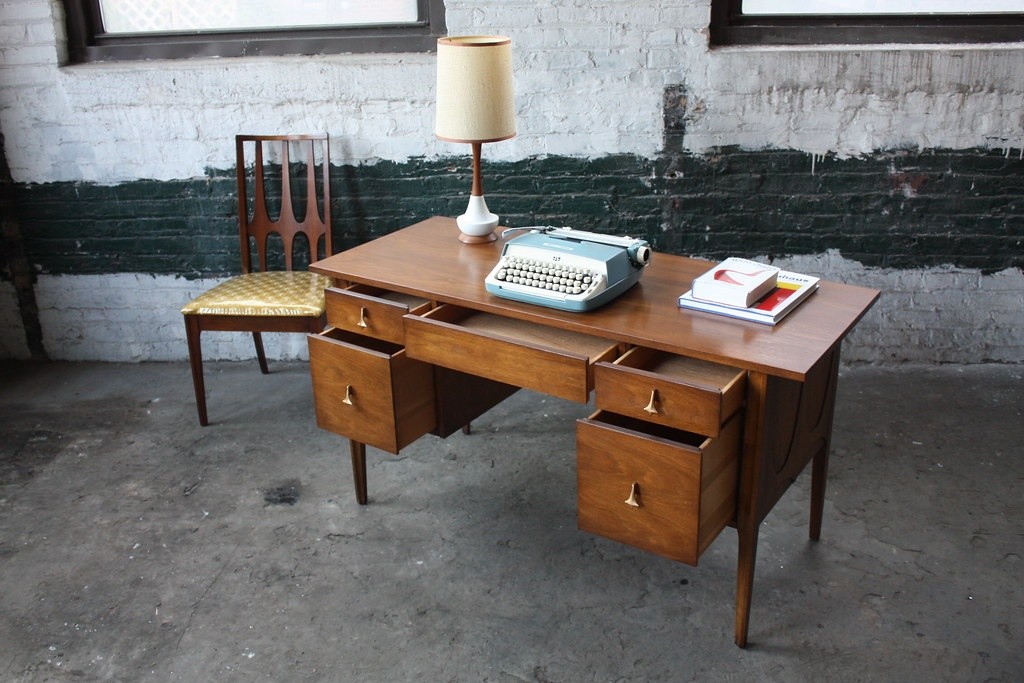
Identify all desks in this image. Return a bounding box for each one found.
[307,216,882,649]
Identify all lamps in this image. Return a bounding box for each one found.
[434,34,517,245]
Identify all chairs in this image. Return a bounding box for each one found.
[180,134,334,428]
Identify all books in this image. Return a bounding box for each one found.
[676,256,822,327]
